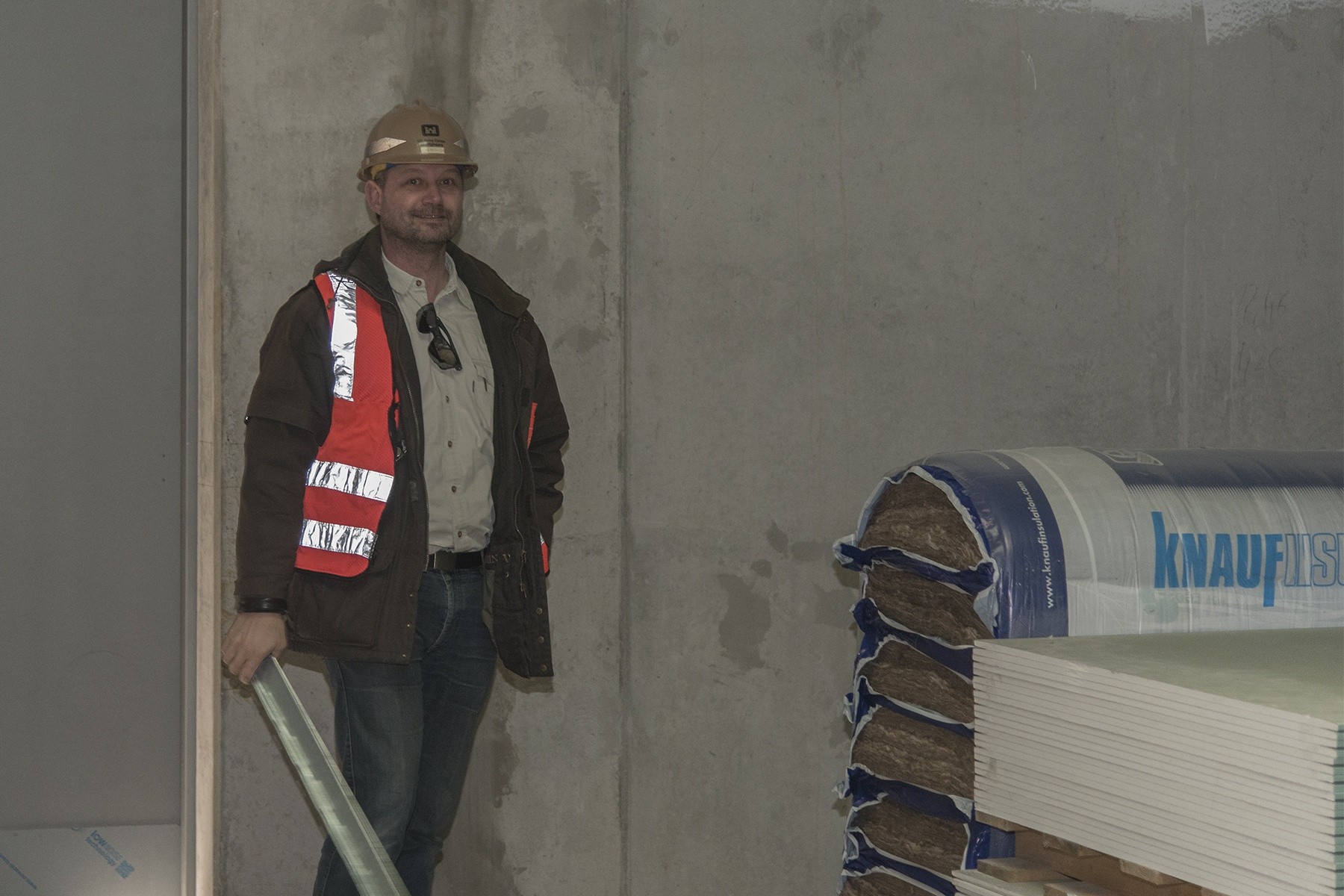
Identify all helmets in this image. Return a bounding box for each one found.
[357,101,478,182]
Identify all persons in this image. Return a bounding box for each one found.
[220,98,569,896]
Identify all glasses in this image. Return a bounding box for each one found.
[416,303,462,371]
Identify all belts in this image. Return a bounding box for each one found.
[427,551,480,570]
